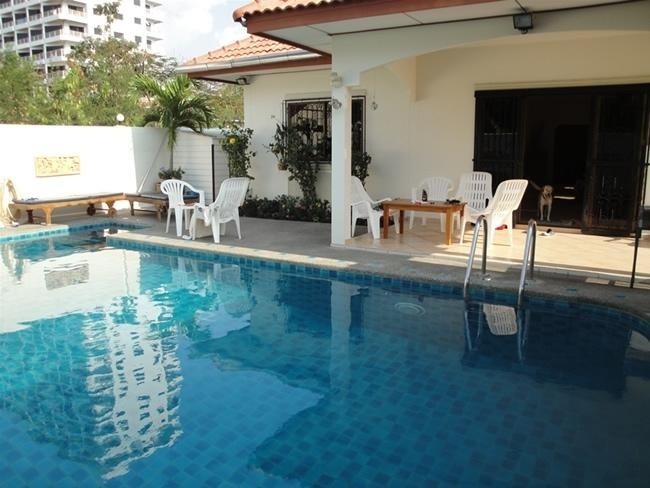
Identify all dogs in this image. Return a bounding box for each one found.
[539,185,554,223]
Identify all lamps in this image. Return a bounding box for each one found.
[513,12,532,34]
[329,72,342,88]
[235,78,248,85]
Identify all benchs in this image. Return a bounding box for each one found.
[14,191,125,223]
[127,192,198,221]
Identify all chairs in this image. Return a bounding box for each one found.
[350,175,400,239]
[169,256,240,293]
[160,179,206,237]
[189,176,249,244]
[409,176,461,232]
[441,171,493,233]
[484,303,517,335]
[459,179,528,244]
